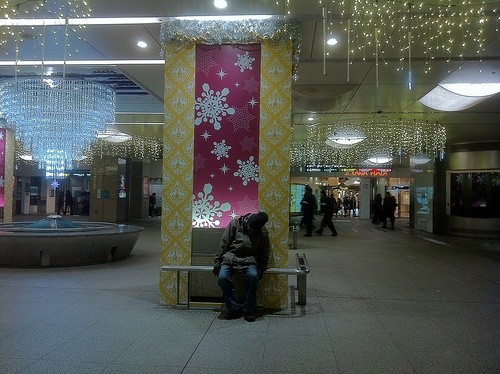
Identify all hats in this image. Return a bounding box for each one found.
[248,212,270,232]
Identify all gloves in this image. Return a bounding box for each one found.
[257,268,263,281]
[213,266,220,278]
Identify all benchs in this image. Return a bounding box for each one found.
[162,252,310,310]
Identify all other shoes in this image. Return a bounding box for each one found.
[218,307,242,320]
[315,230,322,235]
[244,312,256,322]
[331,232,337,236]
[304,234,312,237]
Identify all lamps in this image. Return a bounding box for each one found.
[328,124,431,165]
[419,59,500,112]
[19,151,33,160]
[95,127,131,142]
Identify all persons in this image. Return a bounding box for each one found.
[299,184,397,237]
[213,212,270,322]
[149,193,156,217]
[57,189,90,216]
